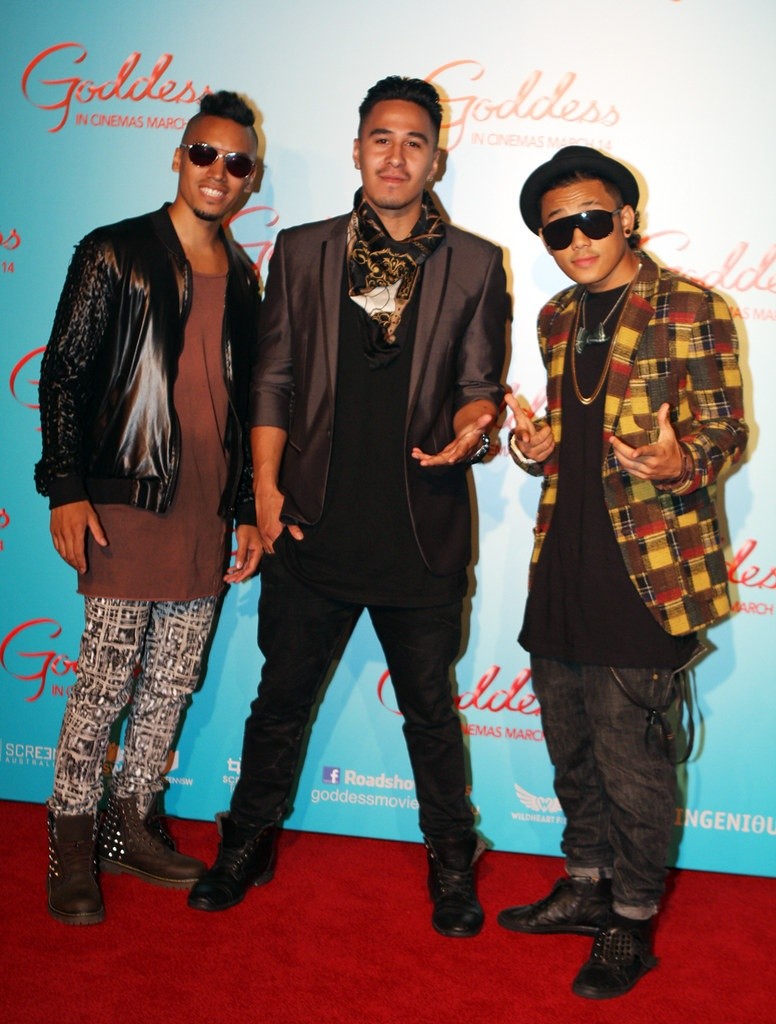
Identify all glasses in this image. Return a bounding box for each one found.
[541,207,623,250]
[179,141,257,179]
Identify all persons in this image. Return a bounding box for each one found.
[495,146,751,1001]
[184,74,512,940]
[31,88,274,933]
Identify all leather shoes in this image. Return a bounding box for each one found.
[571,915,658,1000]
[496,873,608,935]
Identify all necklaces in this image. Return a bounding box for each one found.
[569,252,645,406]
[575,251,643,356]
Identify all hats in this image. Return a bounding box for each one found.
[520,146,640,236]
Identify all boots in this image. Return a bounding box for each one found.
[46,810,105,924]
[424,831,488,937]
[187,809,282,912]
[92,787,212,890]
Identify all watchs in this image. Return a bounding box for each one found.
[466,431,491,466]
